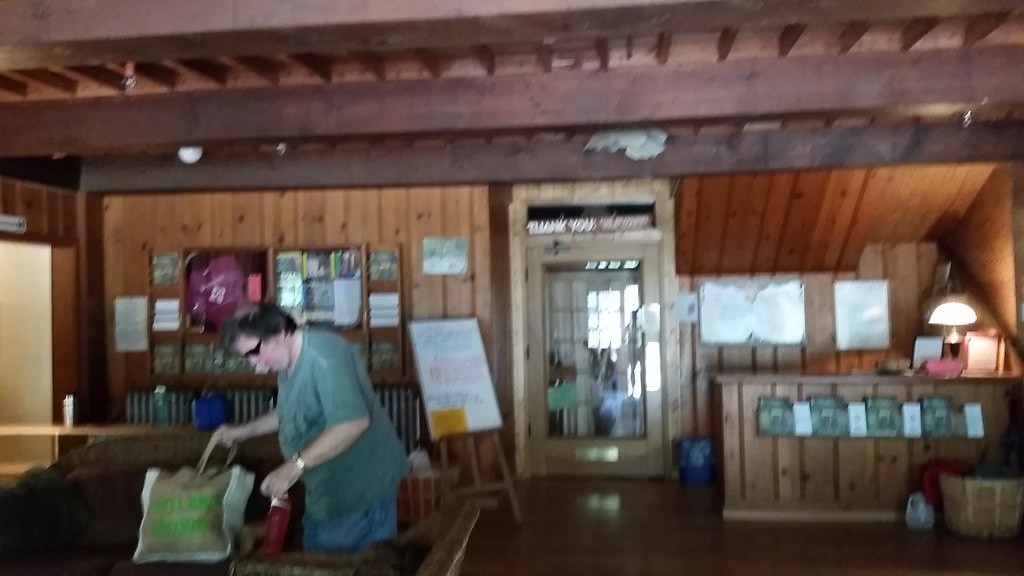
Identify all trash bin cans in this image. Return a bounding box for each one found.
[674,434,723,506]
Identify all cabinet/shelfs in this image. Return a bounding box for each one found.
[146,242,404,388]
[715,374,1022,523]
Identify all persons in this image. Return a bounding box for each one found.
[211,303,413,556]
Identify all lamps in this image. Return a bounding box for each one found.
[928,302,978,363]
[178,146,203,165]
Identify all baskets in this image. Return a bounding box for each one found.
[941,473,1024,538]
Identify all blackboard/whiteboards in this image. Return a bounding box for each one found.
[832,277,892,352]
[697,277,808,347]
[404,316,505,442]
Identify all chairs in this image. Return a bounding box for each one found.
[228,500,480,576]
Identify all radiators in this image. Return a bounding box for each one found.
[125,389,420,456]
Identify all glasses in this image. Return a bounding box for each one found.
[244,337,263,360]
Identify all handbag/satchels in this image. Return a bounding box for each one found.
[134,435,256,564]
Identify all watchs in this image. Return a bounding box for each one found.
[290,451,305,470]
